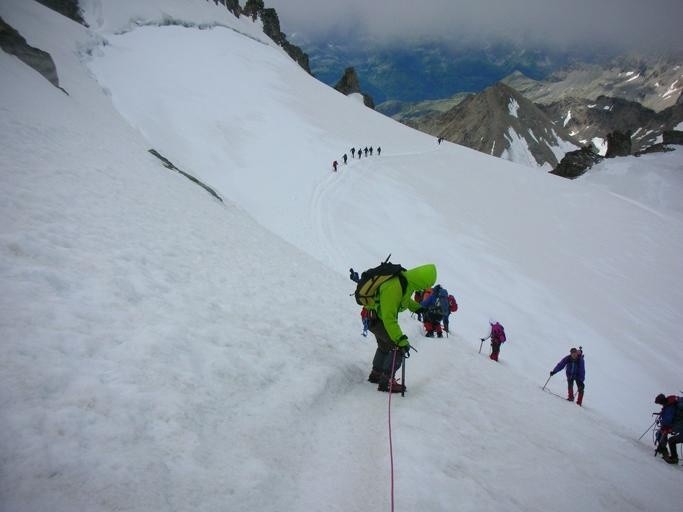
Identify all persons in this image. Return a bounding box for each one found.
[550,348,584,406]
[363,263,437,393]
[479,318,506,361]
[330,147,383,172]
[438,137,441,144]
[648,393,682,464]
[413,286,449,339]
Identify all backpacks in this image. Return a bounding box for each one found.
[354,264,404,306]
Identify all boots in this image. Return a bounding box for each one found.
[660,438,679,464]
[426,331,442,337]
[369,371,406,392]
[567,388,584,405]
[491,353,498,360]
